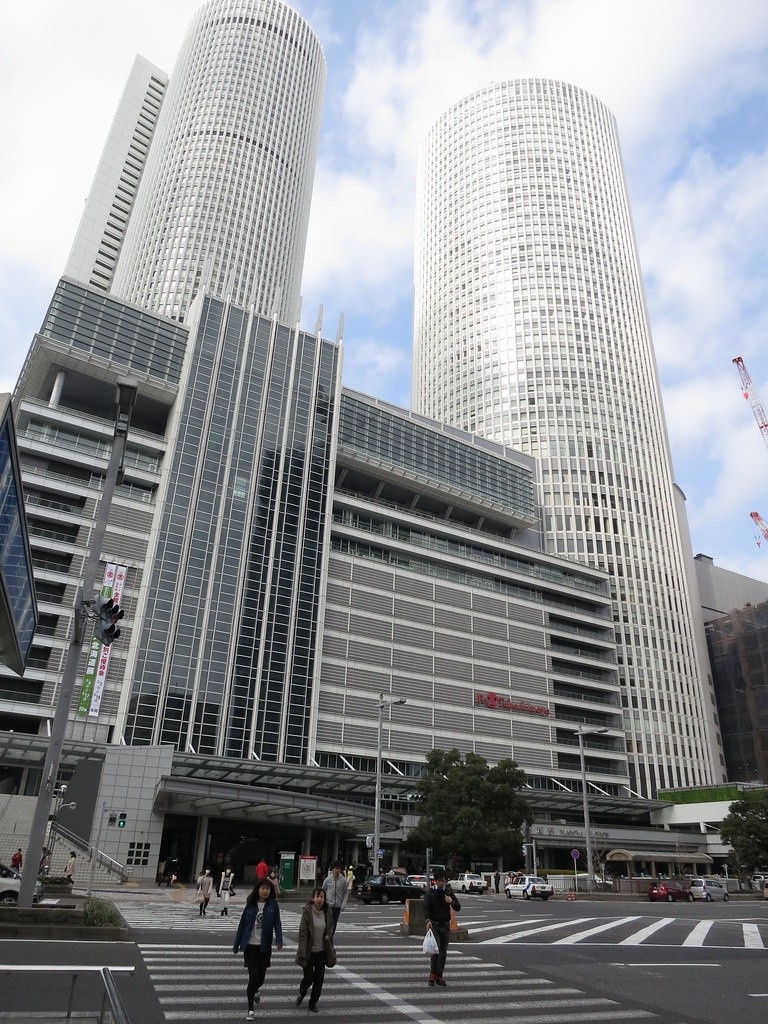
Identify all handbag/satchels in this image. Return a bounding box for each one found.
[192,885,204,904]
[422,929,439,955]
[325,942,337,968]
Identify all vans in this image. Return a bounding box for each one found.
[648,880,694,902]
[689,878,730,901]
[752,874,768,900]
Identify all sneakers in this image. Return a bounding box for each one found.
[247,1011,254,1020]
[255,992,261,1005]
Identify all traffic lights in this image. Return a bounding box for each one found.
[89,593,125,646]
[118,813,127,827]
[521,845,528,856]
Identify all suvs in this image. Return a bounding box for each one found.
[0,860,45,903]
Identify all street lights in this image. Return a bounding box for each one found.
[573,724,611,891]
[366,691,407,876]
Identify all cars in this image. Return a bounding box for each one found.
[505,875,554,901]
[447,873,488,895]
[354,874,423,905]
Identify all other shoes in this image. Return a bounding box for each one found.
[437,976,446,986]
[429,973,436,985]
[221,910,224,916]
[224,907,228,915]
[295,995,304,1006]
[311,1006,319,1012]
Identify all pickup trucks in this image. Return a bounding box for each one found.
[406,875,427,889]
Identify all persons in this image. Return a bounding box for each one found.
[256,857,396,895]
[422,870,461,988]
[323,861,348,939]
[494,869,524,894]
[197,867,213,916]
[64,851,76,880]
[235,877,286,1019]
[218,867,236,919]
[294,886,334,1012]
[156,856,180,888]
[12,846,52,875]
